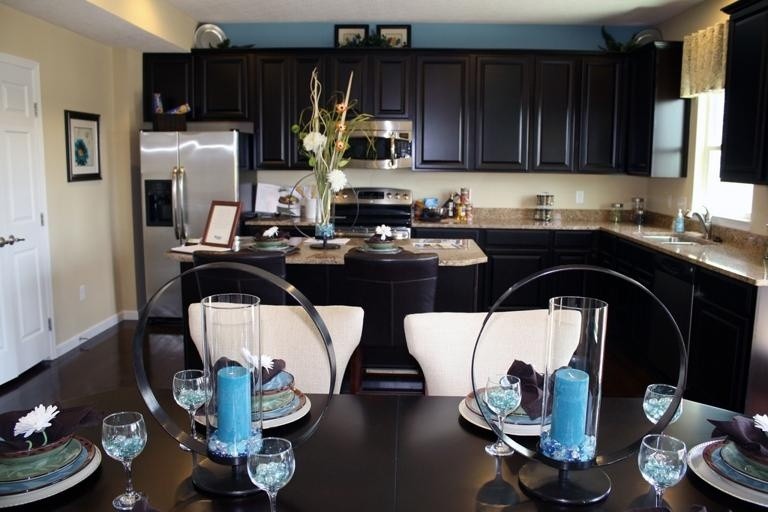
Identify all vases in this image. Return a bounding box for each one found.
[314,181,337,239]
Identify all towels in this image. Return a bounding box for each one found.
[505,359,560,418]
[367,233,398,244]
[0,405,98,449]
[254,228,285,241]
[207,356,287,392]
[709,413,768,458]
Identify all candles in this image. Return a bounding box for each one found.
[214,366,253,456]
[552,367,590,459]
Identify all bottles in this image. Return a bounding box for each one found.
[632,197,645,224]
[414,200,424,221]
[448,194,454,217]
[635,209,644,225]
[671,208,684,233]
[609,202,625,224]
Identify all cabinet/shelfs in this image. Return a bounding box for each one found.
[249,48,328,171]
[625,39,692,180]
[718,0,768,186]
[141,52,195,131]
[476,47,534,172]
[329,47,412,119]
[532,51,624,172]
[411,226,603,313]
[607,234,768,416]
[189,47,258,179]
[413,46,475,172]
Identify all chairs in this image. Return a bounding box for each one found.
[401,307,583,396]
[188,300,366,395]
[339,247,439,393]
[191,249,288,306]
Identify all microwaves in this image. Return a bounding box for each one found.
[325,119,413,170]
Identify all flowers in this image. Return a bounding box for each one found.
[289,64,378,227]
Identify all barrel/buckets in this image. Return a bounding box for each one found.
[663,242,702,245]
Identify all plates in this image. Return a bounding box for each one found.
[357,238,403,254]
[0,410,80,465]
[210,387,306,421]
[702,438,768,493]
[0,437,83,483]
[252,370,294,395]
[0,434,97,496]
[0,443,102,507]
[247,239,296,251]
[719,438,767,484]
[188,396,311,429]
[251,391,296,412]
[465,386,552,425]
[687,435,768,508]
[458,399,552,436]
[482,386,528,414]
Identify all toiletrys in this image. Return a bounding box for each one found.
[675,209,684,233]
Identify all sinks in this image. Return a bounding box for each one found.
[643,236,702,243]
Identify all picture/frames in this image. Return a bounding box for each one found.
[63,109,103,182]
[375,24,412,47]
[201,199,243,248]
[333,23,369,47]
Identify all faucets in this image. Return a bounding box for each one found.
[684,204,711,239]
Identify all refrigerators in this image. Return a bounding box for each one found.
[137,129,258,323]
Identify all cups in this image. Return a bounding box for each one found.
[173,369,214,453]
[643,384,684,434]
[539,295,609,464]
[200,293,264,460]
[638,435,687,511]
[100,411,148,510]
[247,438,297,512]
[484,374,522,456]
